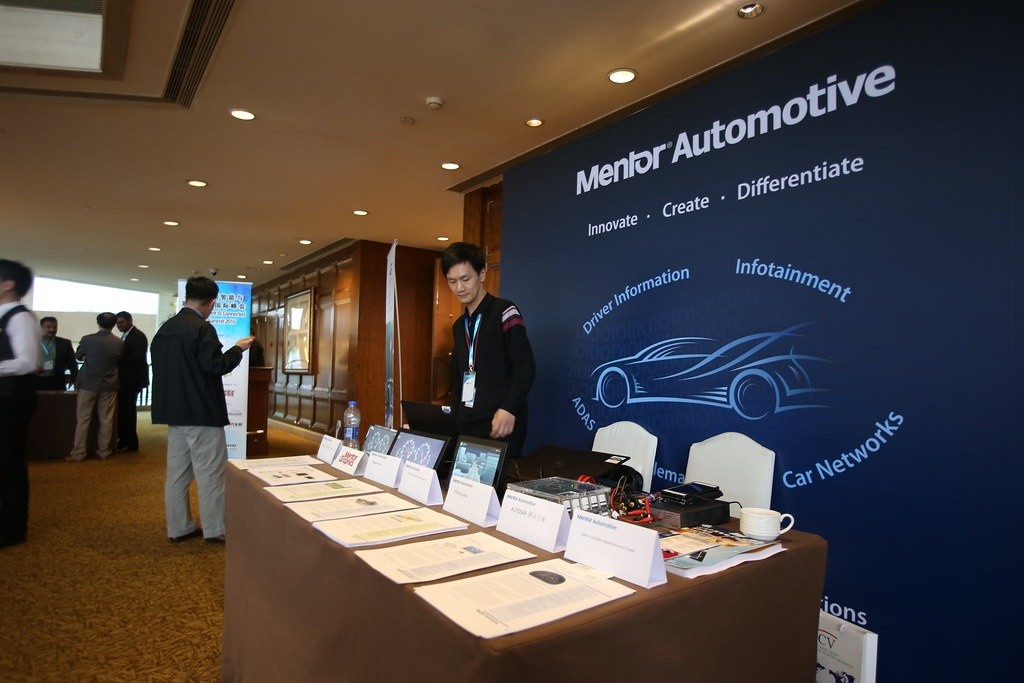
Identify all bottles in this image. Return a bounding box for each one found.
[343,400,361,451]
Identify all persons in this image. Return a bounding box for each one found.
[115,311,150,452]
[250,340,266,368]
[0,259,43,548]
[38,317,79,390]
[440,242,537,461]
[151,277,256,543]
[65,313,125,461]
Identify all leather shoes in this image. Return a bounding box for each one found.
[118,445,138,453]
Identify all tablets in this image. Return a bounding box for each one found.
[447,433,509,489]
[360,423,399,455]
[388,428,452,471]
[662,480,720,498]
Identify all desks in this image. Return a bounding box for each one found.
[224,455,827,683]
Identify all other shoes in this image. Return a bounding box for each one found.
[0,535,25,548]
[65,456,75,462]
[170,526,204,542]
[100,457,108,461]
[205,535,226,544]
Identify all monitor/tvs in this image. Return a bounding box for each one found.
[401,400,465,461]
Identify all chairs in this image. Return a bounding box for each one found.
[683,432,775,524]
[590,421,658,493]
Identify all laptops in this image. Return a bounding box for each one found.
[507,443,629,485]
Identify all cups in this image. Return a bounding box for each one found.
[738,507,795,543]
